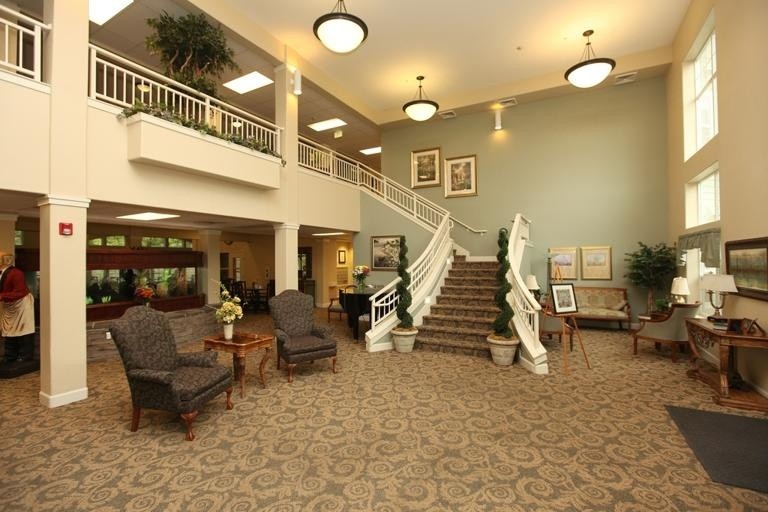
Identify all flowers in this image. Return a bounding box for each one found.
[209,276,250,324]
[351,263,371,286]
[132,287,155,305]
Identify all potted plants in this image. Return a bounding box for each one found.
[619,235,687,330]
[485,226,521,368]
[391,231,420,354]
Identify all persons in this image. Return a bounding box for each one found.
[85,267,197,304]
[0,251,35,363]
[454,162,468,182]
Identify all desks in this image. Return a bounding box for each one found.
[684,317,767,412]
[202,331,276,398]
[336,286,400,344]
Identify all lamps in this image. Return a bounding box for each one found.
[564,27,617,91]
[282,63,306,101]
[697,271,739,324]
[396,71,445,125]
[524,273,542,300]
[311,3,376,59]
[489,101,507,135]
[135,79,150,95]
[670,275,691,298]
[550,282,579,315]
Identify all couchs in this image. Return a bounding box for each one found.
[556,286,636,335]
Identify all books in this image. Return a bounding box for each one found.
[671,302,701,308]
[712,321,728,331]
[636,313,667,321]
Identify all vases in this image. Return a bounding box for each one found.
[357,277,364,288]
[221,322,235,341]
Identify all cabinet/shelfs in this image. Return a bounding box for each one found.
[539,307,592,379]
[268,279,316,307]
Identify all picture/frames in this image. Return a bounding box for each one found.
[722,236,768,303]
[547,245,578,280]
[368,233,406,270]
[443,151,480,200]
[579,245,612,281]
[408,147,442,191]
[337,248,347,265]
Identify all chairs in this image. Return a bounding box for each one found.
[327,284,358,329]
[266,289,340,382]
[225,278,276,317]
[109,302,236,440]
[630,300,702,363]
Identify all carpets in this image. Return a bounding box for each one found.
[663,397,768,496]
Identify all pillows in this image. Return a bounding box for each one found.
[608,298,631,311]
